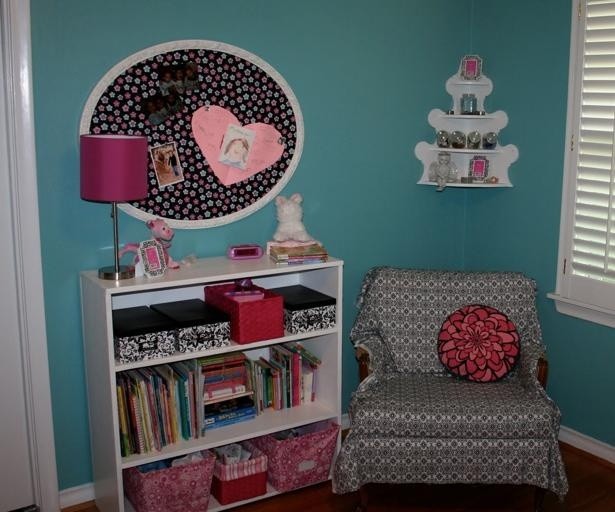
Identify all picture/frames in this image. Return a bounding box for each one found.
[80,37,306,231]
[133,239,167,278]
[466,153,491,185]
[458,52,483,81]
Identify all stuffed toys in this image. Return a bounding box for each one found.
[273,192,313,243]
[118,218,179,269]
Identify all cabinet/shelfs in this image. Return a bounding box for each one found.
[412,64,522,190]
[69,235,350,511]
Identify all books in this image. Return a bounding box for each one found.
[117,342,322,457]
[270,245,328,265]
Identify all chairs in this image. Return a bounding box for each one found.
[333,260,572,511]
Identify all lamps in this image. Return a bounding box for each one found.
[78,129,152,283]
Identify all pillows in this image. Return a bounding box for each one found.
[436,300,522,384]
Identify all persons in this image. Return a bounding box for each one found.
[435,152,452,192]
[222,137,248,169]
[145,67,199,125]
[154,150,180,177]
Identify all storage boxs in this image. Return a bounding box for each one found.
[208,441,271,507]
[111,276,337,366]
[253,418,344,494]
[121,452,217,511]
